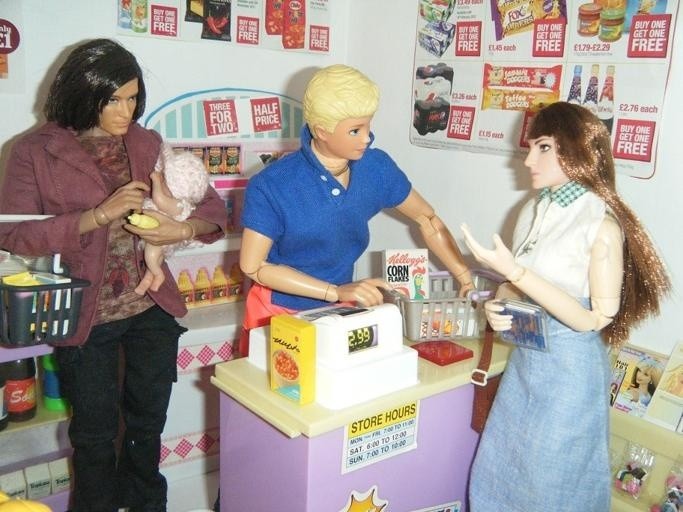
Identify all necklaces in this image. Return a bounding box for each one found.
[334,162,349,177]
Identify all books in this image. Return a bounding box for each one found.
[608,341,668,419]
[382,248,431,300]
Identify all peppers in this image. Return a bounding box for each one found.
[207,17,227,33]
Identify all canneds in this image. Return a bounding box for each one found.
[192,148,238,174]
[577,3,603,37]
[598,7,625,42]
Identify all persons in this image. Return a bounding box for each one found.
[609,382,617,406]
[460,102,669,512]
[239,65,477,357]
[625,357,662,403]
